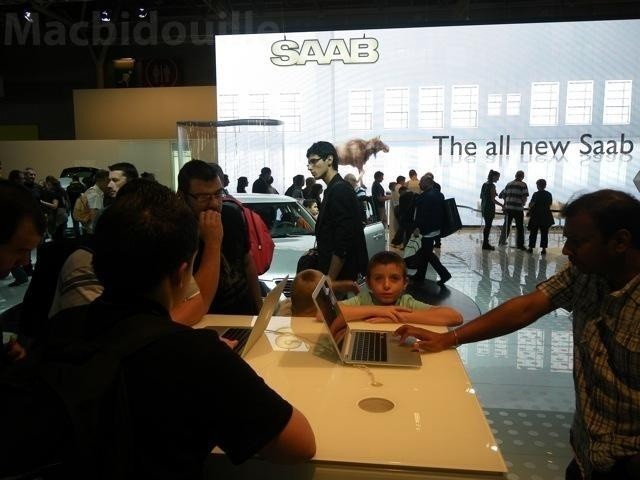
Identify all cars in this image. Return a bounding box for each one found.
[220,192,388,303]
[58,166,98,191]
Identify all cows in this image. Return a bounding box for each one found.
[333,134,390,190]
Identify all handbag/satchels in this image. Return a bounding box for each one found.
[440,199,462,239]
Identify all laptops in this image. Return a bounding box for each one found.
[311,274,423,368]
[204,274,290,359]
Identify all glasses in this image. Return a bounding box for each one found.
[188,191,226,203]
[307,158,321,166]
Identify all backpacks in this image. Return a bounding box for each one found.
[222,193,275,276]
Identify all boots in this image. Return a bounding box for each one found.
[482,239,495,251]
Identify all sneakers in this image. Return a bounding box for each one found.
[437,273,451,285]
[406,274,423,285]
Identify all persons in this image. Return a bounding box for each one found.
[526,179,555,255]
[481,169,503,251]
[0,141,464,479]
[393,188,639,480]
[498,171,529,250]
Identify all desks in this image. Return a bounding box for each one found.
[191,315,509,480]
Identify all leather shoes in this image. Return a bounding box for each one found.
[517,244,546,254]
